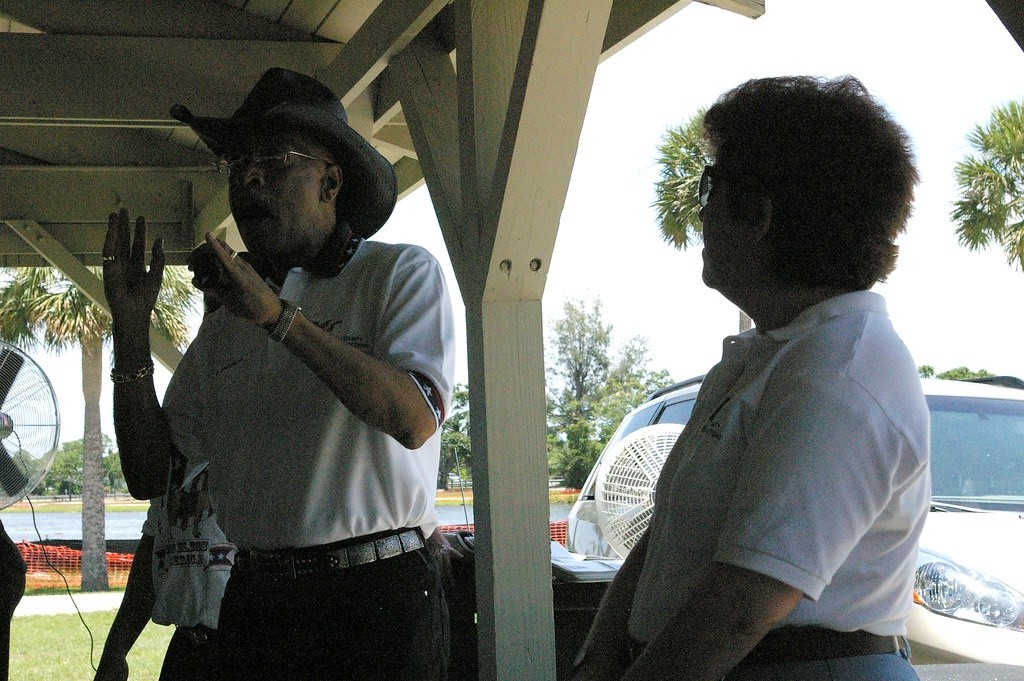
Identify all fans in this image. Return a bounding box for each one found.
[594,423,686,559]
[0,339,61,511]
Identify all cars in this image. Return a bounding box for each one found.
[564,379,1024,665]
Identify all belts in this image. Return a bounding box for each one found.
[233,529,425,581]
[619,633,897,668]
[177,626,214,648]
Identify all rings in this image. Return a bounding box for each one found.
[103,256,114,260]
[230,250,239,258]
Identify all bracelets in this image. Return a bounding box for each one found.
[270,304,298,342]
[110,366,153,383]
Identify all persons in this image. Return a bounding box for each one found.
[103,69,454,680]
[92,463,238,680]
[0,519,30,681]
[568,74,932,681]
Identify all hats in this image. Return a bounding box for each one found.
[169,67,397,239]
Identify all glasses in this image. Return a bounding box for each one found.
[210,141,336,176]
[699,165,749,200]
